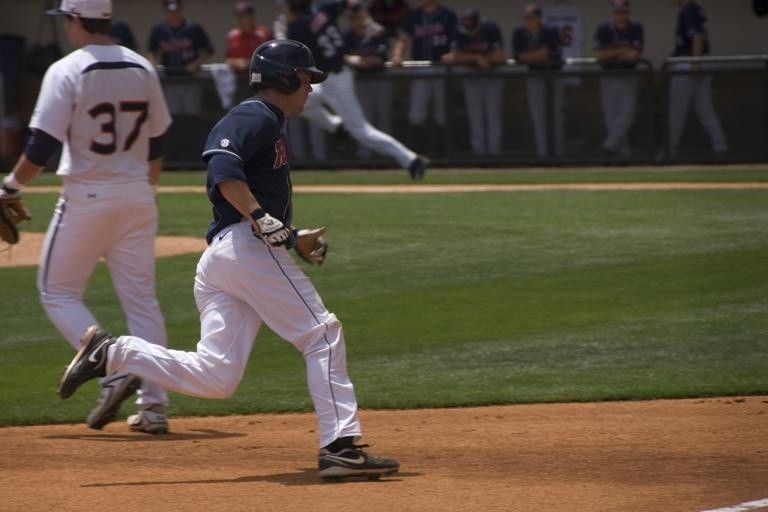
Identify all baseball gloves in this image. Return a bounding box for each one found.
[0,187,31,244]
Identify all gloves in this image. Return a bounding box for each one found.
[294,225,328,266]
[250,208,297,249]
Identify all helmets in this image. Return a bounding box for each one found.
[248,39,327,94]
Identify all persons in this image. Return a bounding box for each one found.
[1,2,730,180]
[56,38,400,482]
[0,1,171,434]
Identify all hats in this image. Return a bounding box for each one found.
[45,0,114,20]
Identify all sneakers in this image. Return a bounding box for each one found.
[409,156,425,179]
[88,371,142,430]
[318,444,399,481]
[127,404,168,434]
[56,323,118,399]
